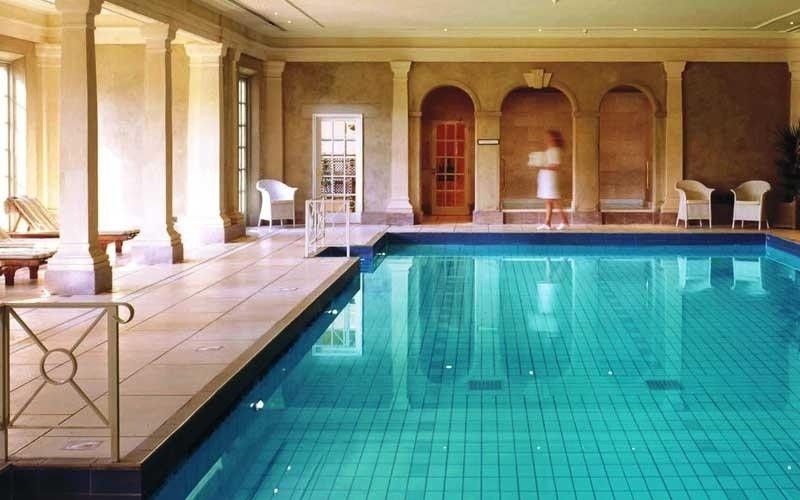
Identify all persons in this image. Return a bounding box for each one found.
[534,128,570,231]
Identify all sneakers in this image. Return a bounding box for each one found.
[537,223,568,230]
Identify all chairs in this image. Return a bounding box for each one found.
[676,256,771,295]
[0,194,140,286]
[255,179,299,229]
[673,179,771,232]
